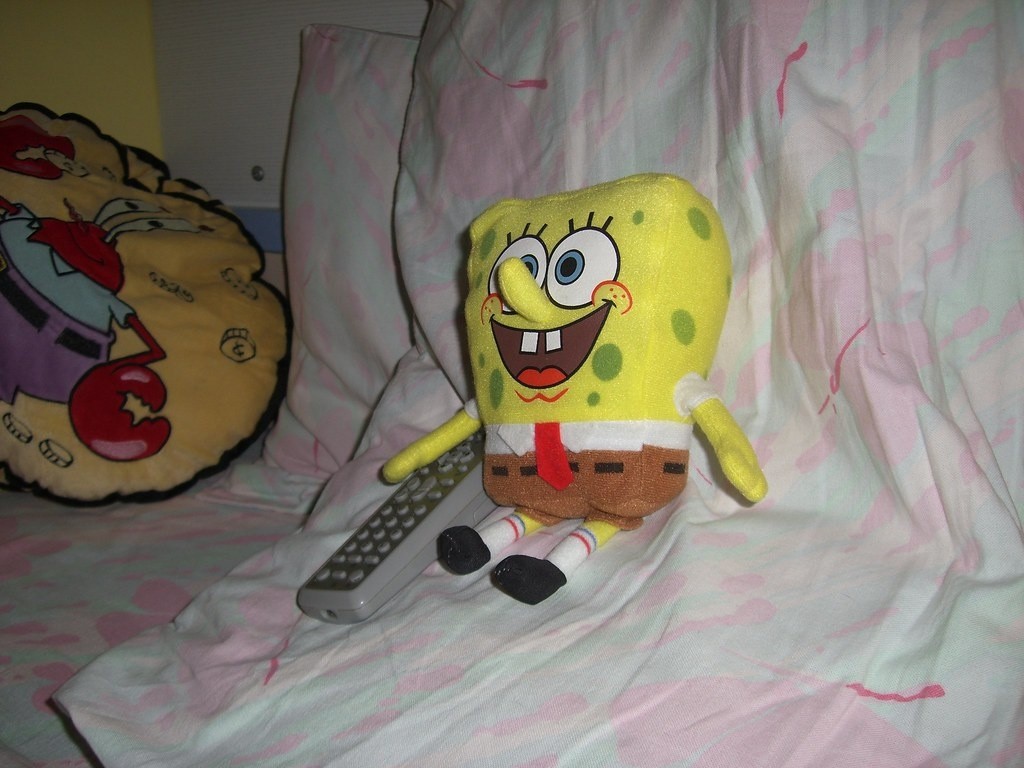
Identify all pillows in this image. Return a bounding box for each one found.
[0,101,294,508]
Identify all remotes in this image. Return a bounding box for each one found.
[295,429,499,624]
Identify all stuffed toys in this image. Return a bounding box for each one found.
[382,175,769,604]
[0,101,291,505]
